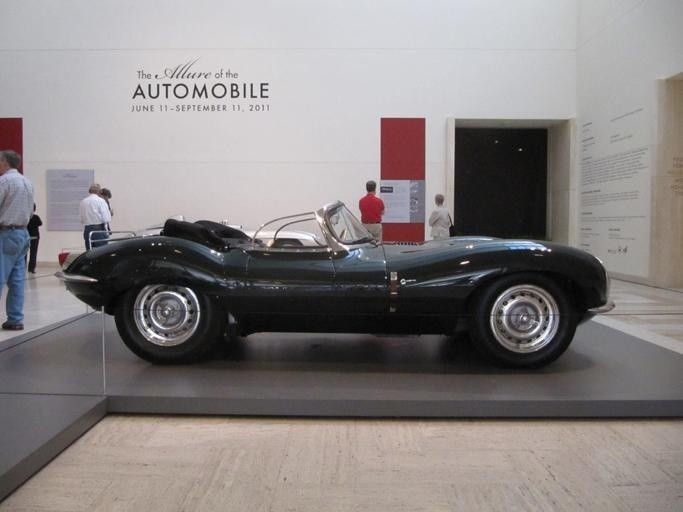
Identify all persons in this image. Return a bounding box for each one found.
[359,180,385,243]
[25,203,42,274]
[79,182,114,251]
[0,149,34,330]
[428,194,451,240]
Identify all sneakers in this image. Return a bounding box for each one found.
[2,321,24,331]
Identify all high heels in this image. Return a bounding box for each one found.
[28,270,36,274]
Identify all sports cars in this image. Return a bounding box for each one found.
[124,213,319,247]
[51,200,617,367]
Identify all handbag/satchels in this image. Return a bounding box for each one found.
[448,214,456,237]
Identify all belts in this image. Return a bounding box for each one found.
[361,222,380,224]
[0,225,25,230]
